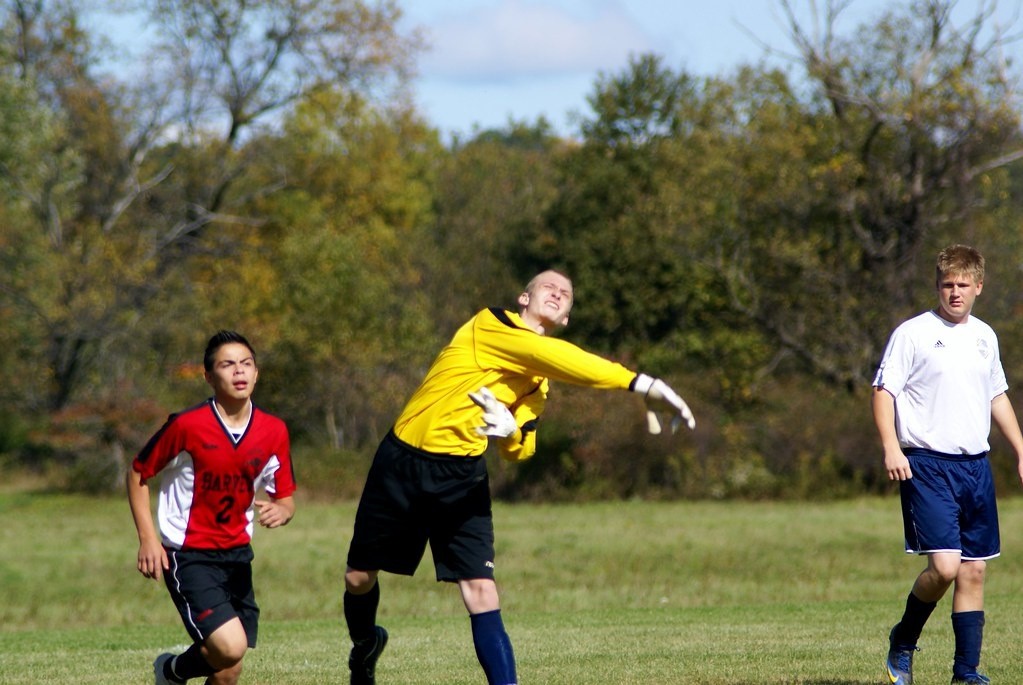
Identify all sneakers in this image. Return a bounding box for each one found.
[349,626,388,685]
[152,653,186,685]
[951,674,991,685]
[886,622,921,685]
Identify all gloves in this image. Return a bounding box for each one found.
[468,387,517,439]
[628,372,696,435]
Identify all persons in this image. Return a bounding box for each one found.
[129,330,297,685]
[870,243,1023,685]
[343,269,695,685]
